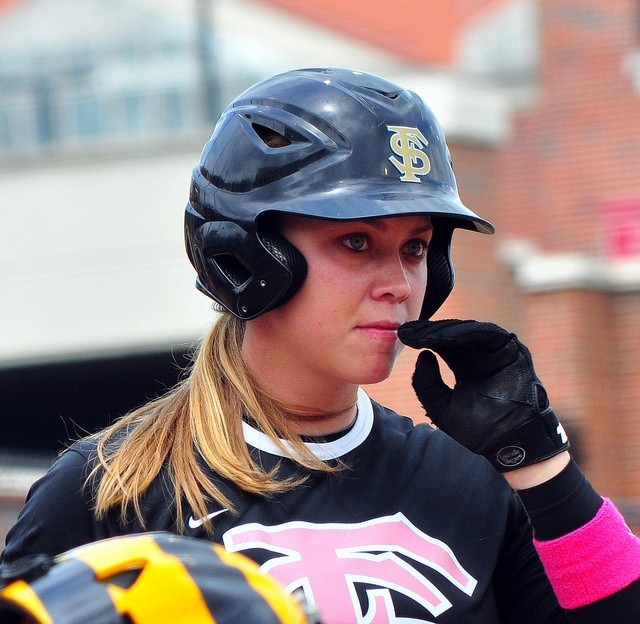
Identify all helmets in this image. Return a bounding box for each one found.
[3,531,309,624]
[182,66,494,329]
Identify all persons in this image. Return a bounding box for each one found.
[0,70,640,623]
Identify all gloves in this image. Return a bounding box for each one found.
[396,319,568,468]
